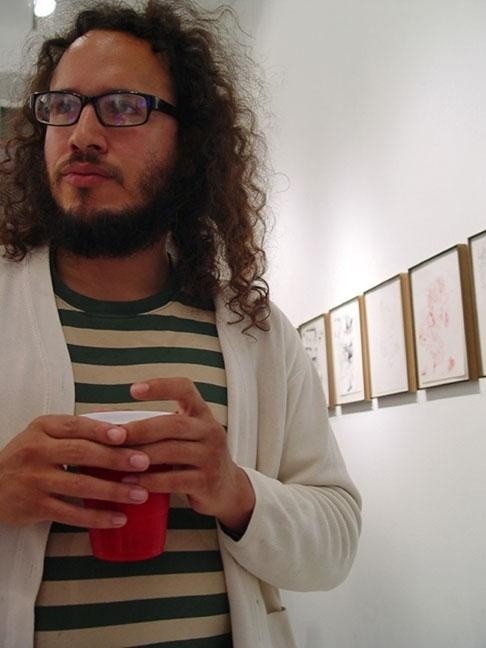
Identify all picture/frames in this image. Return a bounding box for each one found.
[295,223,485,412]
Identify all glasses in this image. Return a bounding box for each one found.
[29,87,184,127]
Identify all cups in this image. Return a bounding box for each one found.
[67,406,182,564]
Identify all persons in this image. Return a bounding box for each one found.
[2,0,363,648]
